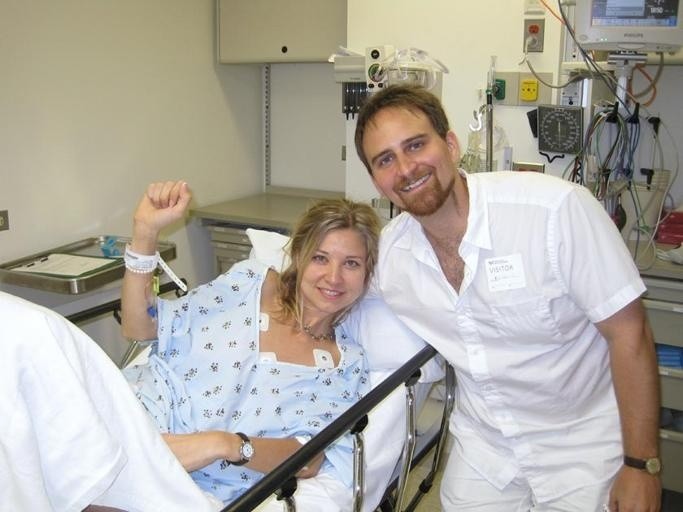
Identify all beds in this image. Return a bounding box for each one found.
[68,278,454,512]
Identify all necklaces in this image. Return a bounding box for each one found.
[299,321,339,342]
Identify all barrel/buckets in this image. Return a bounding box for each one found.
[619,185,664,241]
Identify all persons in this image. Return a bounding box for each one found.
[122,179,382,504]
[351,83,663,512]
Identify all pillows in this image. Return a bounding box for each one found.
[244,229,447,382]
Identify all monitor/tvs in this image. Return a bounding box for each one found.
[572,0,683,54]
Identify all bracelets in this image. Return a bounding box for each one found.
[124,242,162,274]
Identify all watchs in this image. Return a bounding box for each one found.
[226,433,255,466]
[623,454,662,475]
